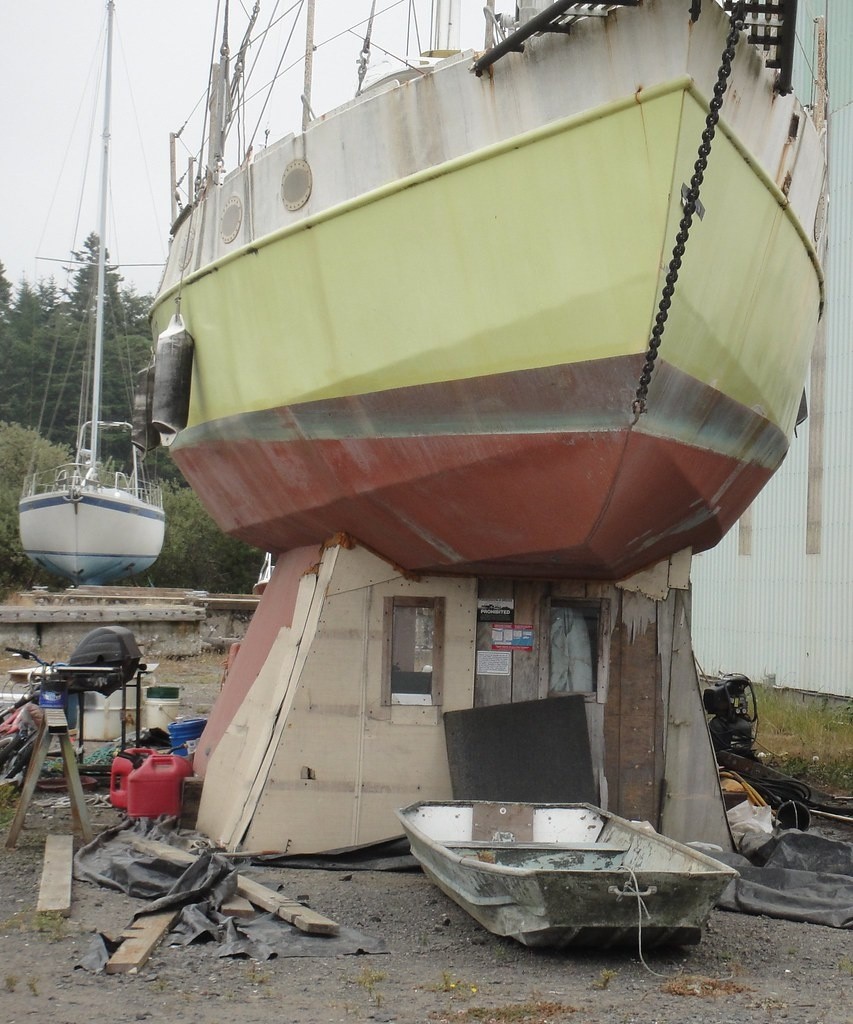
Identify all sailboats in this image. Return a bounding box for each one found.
[19,2,164,588]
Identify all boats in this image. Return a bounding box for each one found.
[396,798,738,946]
[164,0,827,581]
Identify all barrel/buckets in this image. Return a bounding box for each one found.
[110,744,188,809]
[168,718,207,760]
[116,752,193,819]
[145,697,180,735]
[39,680,68,709]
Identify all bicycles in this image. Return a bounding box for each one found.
[0,646,70,780]
